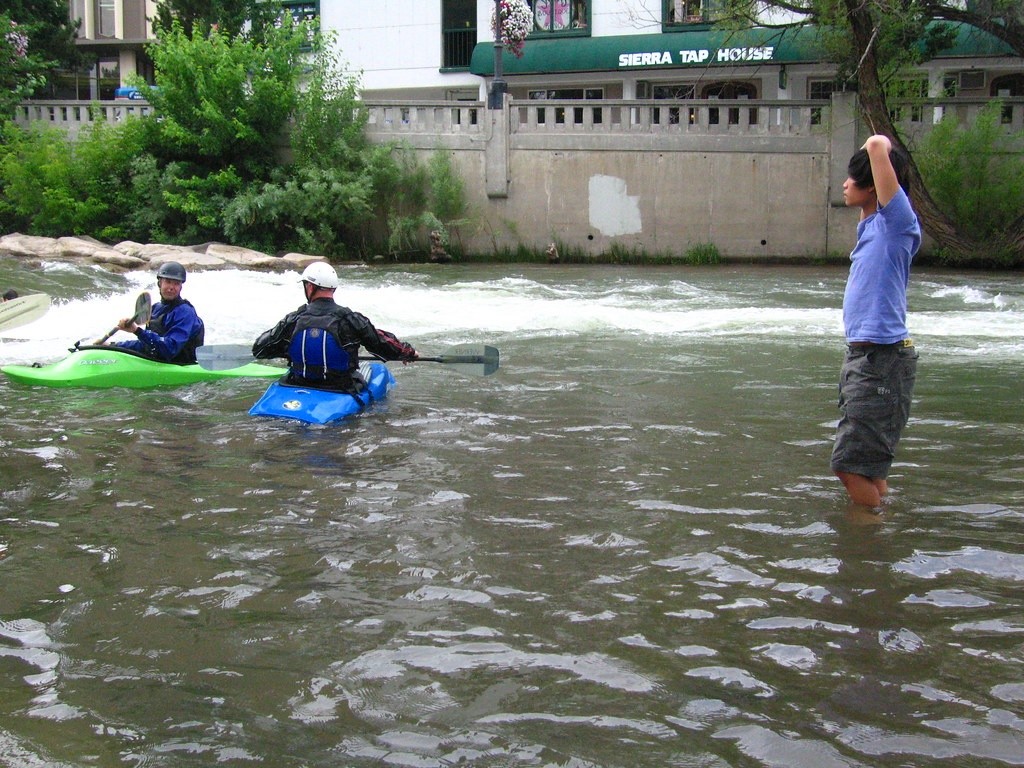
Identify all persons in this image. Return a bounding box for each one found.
[253,261,419,389]
[0,289,18,302]
[831,135,920,502]
[93,260,205,364]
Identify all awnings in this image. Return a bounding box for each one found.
[470,16,1024,75]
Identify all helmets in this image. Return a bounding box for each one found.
[297,261,339,288]
[157,261,186,283]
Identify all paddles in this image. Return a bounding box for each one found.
[0,294,51,331]
[95,293,151,346]
[356,343,499,374]
